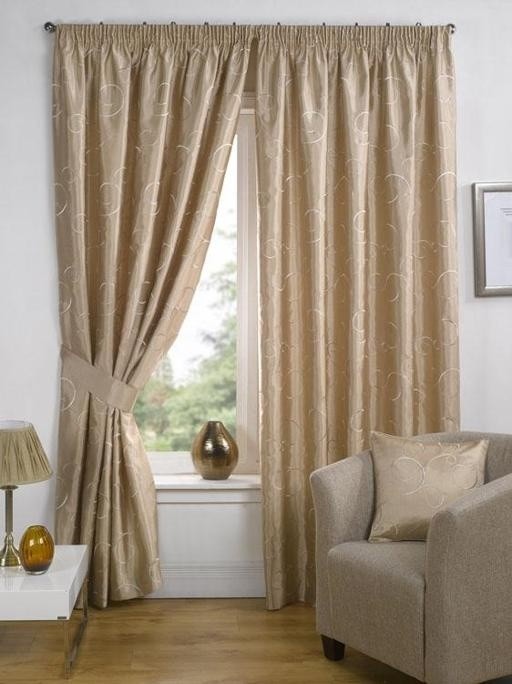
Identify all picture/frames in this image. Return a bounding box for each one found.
[471,182,512,298]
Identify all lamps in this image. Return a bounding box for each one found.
[0,418,54,568]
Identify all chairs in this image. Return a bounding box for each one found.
[308,429,512,684]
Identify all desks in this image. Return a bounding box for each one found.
[0,544,92,684]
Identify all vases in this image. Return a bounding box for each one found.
[190,420,240,481]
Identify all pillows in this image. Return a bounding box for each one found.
[366,429,489,543]
